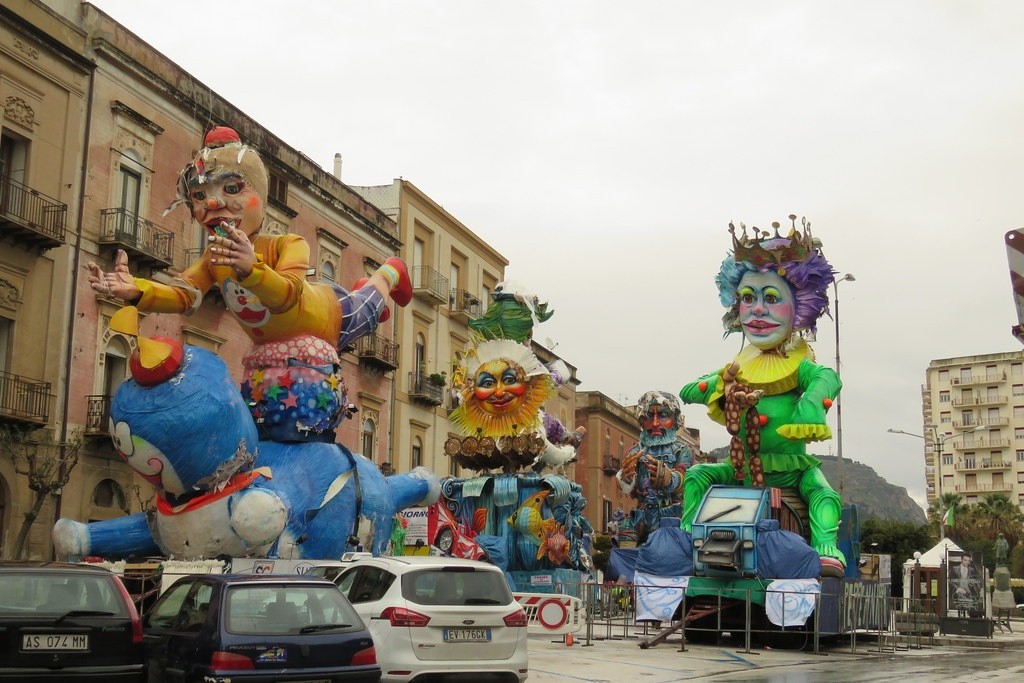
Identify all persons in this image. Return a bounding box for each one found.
[87,129,412,354]
[677,214,848,578]
[428,293,594,573]
[950,553,980,597]
[602,389,694,582]
[991,533,1009,567]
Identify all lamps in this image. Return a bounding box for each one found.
[911,547,924,564]
[940,552,947,562]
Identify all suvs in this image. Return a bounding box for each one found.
[309,554,529,683]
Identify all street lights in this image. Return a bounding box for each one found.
[887,424,989,543]
[828,268,856,506]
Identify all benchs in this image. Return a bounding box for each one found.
[990,608,1013,634]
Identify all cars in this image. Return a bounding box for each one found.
[257,549,374,612]
[141,572,384,683]
[0,554,149,683]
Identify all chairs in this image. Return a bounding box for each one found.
[461,579,484,598]
[434,577,459,598]
[263,600,300,626]
[36,583,81,611]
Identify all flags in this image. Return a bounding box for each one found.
[942,505,956,527]
